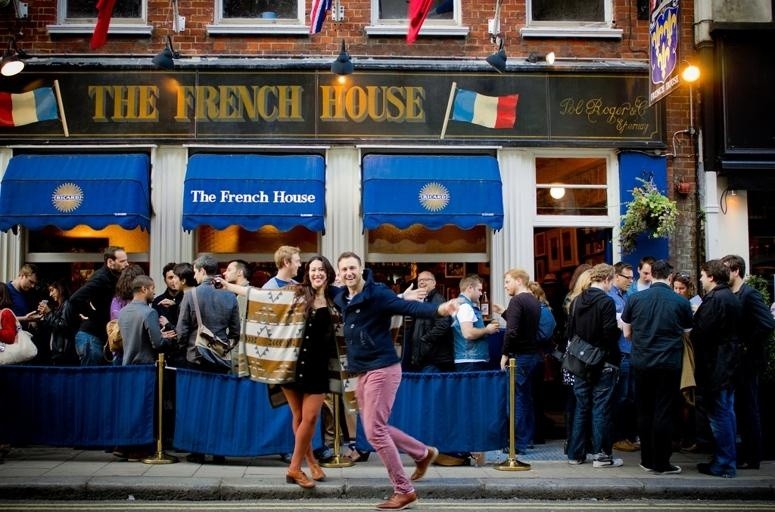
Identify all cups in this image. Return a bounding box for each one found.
[492,320,500,330]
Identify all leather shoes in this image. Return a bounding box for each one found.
[376,491,417,511]
[410,446,439,481]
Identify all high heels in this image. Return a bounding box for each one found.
[306,463,325,480]
[287,467,316,489]
[343,442,370,462]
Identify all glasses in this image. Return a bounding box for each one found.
[618,272,635,280]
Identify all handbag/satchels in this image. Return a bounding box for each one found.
[195,324,233,368]
[106,319,123,351]
[0,329,39,364]
[561,336,608,382]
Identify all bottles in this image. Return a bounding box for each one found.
[480,292,489,320]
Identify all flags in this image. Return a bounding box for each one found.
[0,87,58,127]
[90,0,116,50]
[309,0,332,36]
[406,1,432,44]
[453,86,521,130]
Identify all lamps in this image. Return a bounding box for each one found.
[528,51,554,65]
[674,60,700,82]
[549,159,565,199]
[0,37,29,77]
[331,38,354,75]
[151,35,179,68]
[485,36,506,76]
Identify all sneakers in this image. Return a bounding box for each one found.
[568,438,682,475]
[697,462,713,474]
[283,454,292,463]
[185,452,225,463]
[737,460,760,471]
[319,450,332,460]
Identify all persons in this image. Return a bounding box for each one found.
[0,263,70,366]
[566,255,775,479]
[217,250,459,511]
[71,246,557,453]
